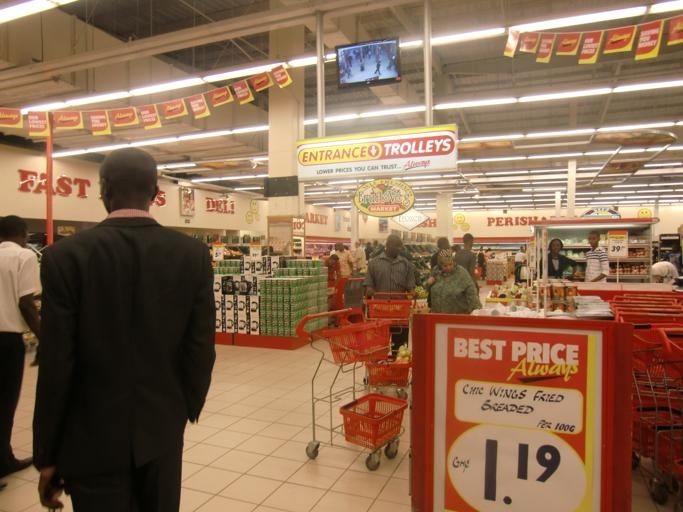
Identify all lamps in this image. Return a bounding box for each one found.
[0,0,682,209]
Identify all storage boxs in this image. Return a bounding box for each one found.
[240,256,255,275]
[212,274,222,295]
[246,295,259,315]
[221,295,234,315]
[234,275,257,296]
[249,245,267,256]
[321,318,392,365]
[266,245,274,256]
[234,295,247,316]
[222,314,236,334]
[364,359,411,388]
[221,275,236,295]
[245,315,260,336]
[214,294,222,310]
[365,299,412,328]
[340,392,410,450]
[630,347,683,481]
[271,255,285,269]
[234,314,248,334]
[215,310,222,332]
[255,256,271,275]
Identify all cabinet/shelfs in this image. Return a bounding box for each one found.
[534,215,660,287]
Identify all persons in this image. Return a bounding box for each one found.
[394,57,397,72]
[487,248,491,259]
[385,58,392,70]
[483,250,488,259]
[421,249,481,315]
[452,244,460,254]
[540,239,569,278]
[477,248,486,280]
[450,246,456,258]
[429,236,449,269]
[448,246,451,253]
[376,46,382,62]
[269,246,276,256]
[513,245,527,283]
[344,64,353,79]
[31,147,215,511]
[319,254,340,288]
[0,215,41,489]
[343,245,348,251]
[584,231,609,283]
[327,244,336,255]
[364,242,372,261]
[374,61,382,74]
[651,261,678,284]
[358,59,364,72]
[371,240,378,250]
[347,55,352,67]
[454,233,476,282]
[329,242,352,277]
[367,48,372,60]
[351,240,365,272]
[360,234,424,362]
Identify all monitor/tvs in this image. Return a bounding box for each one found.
[335,36,402,88]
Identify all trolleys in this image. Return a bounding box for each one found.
[328,277,349,328]
[363,291,417,400]
[609,291,683,512]
[296,308,408,471]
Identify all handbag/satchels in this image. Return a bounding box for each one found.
[427,267,435,307]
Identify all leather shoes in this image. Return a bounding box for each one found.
[0,481,9,491]
[0,457,35,479]
